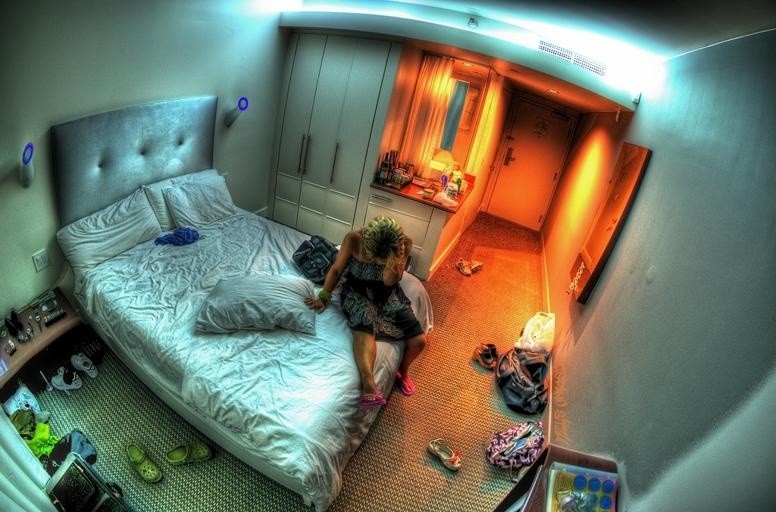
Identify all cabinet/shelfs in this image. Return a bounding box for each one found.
[267,27,467,281]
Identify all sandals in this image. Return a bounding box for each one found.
[428,438,462,472]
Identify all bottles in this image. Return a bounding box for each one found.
[377,148,398,184]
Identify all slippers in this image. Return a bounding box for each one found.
[473,348,497,370]
[126,442,163,483]
[396,370,416,396]
[362,387,387,408]
[165,441,212,466]
[479,343,500,362]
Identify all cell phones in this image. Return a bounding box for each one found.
[43,308,66,327]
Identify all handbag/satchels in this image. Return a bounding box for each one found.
[495,348,548,414]
[292,234,339,284]
[486,419,545,469]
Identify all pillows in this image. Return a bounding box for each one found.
[55,168,237,276]
[196,274,316,335]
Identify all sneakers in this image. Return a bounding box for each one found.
[51,366,83,390]
[470,261,484,272]
[70,353,100,379]
[455,258,472,275]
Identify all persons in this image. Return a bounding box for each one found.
[303,215,427,407]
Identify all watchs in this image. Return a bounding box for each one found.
[34,311,42,334]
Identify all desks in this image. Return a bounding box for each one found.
[0,289,83,389]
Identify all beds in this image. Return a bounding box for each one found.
[50,94,429,509]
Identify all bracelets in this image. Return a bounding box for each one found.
[317,289,332,303]
